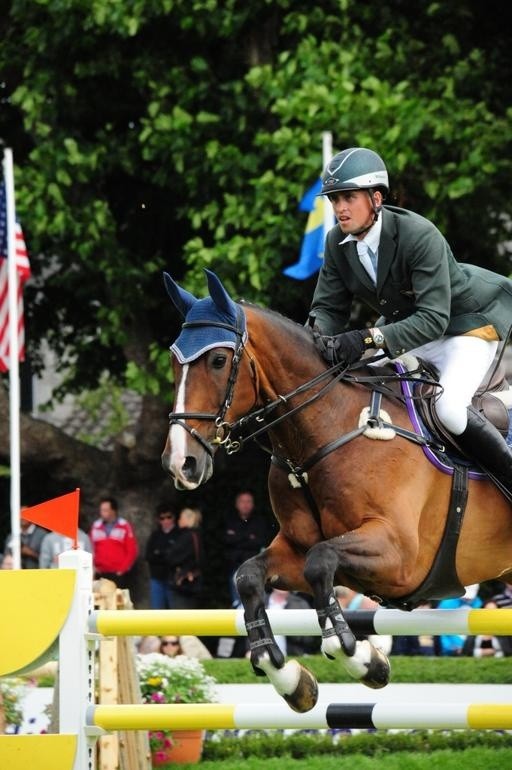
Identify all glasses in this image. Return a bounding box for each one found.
[162,641,179,646]
[160,513,174,519]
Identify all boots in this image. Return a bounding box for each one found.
[448,406,512,492]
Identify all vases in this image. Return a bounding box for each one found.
[147,728,207,770]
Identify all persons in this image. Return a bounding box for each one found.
[302,148,512,506]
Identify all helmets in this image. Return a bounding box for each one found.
[315,147,390,196]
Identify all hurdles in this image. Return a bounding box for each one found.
[50,549,512,770]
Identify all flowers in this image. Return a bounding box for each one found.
[129,647,220,763]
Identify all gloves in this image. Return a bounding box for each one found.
[330,328,373,366]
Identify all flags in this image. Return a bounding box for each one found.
[0,159,32,375]
[283,173,339,280]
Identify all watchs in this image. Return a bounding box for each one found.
[372,326,385,349]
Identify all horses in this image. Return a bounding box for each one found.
[161,268,512,715]
